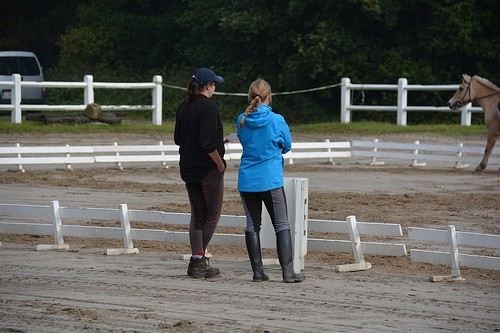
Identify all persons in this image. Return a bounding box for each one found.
[237,79,306,283]
[173,68,227,278]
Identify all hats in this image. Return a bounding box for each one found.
[192,68,224,84]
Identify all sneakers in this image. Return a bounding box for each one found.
[187,255,220,280]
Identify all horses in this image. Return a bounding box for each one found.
[447,74,500,173]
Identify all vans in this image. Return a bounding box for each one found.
[0,51,46,106]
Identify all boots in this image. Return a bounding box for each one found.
[245,229,269,281]
[276,229,305,282]
